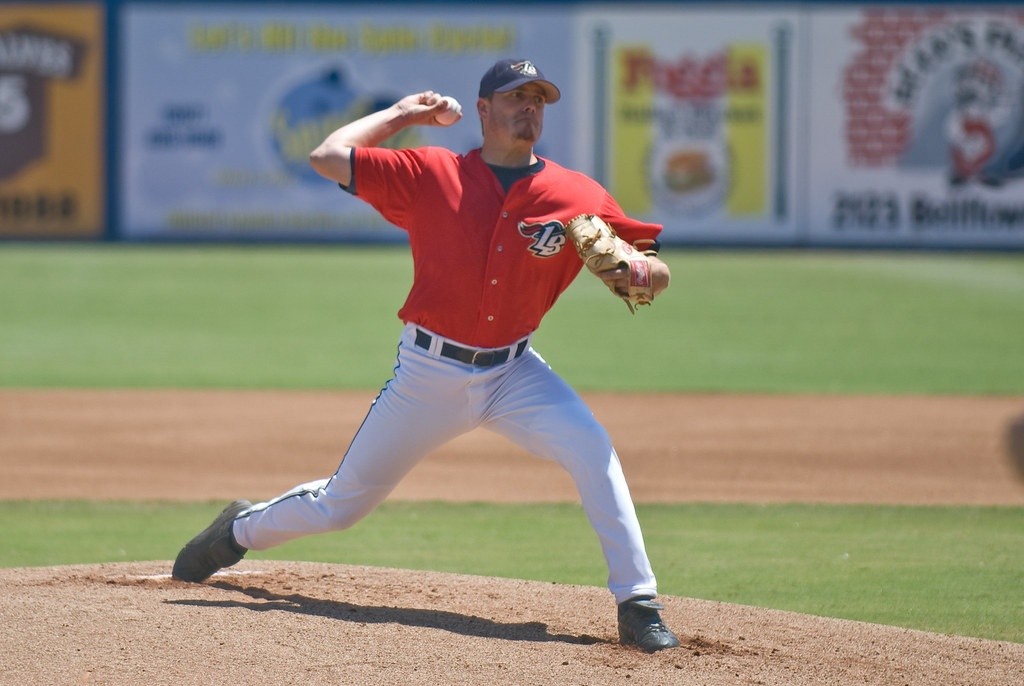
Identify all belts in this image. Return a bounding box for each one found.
[414,329,528,369]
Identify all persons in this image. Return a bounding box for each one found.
[171,58,681,655]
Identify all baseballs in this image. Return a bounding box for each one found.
[433,95,462,128]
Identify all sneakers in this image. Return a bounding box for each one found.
[171,498,254,583]
[617,600,680,654]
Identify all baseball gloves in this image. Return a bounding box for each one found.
[563,211,659,318]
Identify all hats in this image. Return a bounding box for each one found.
[479,58,561,105]
